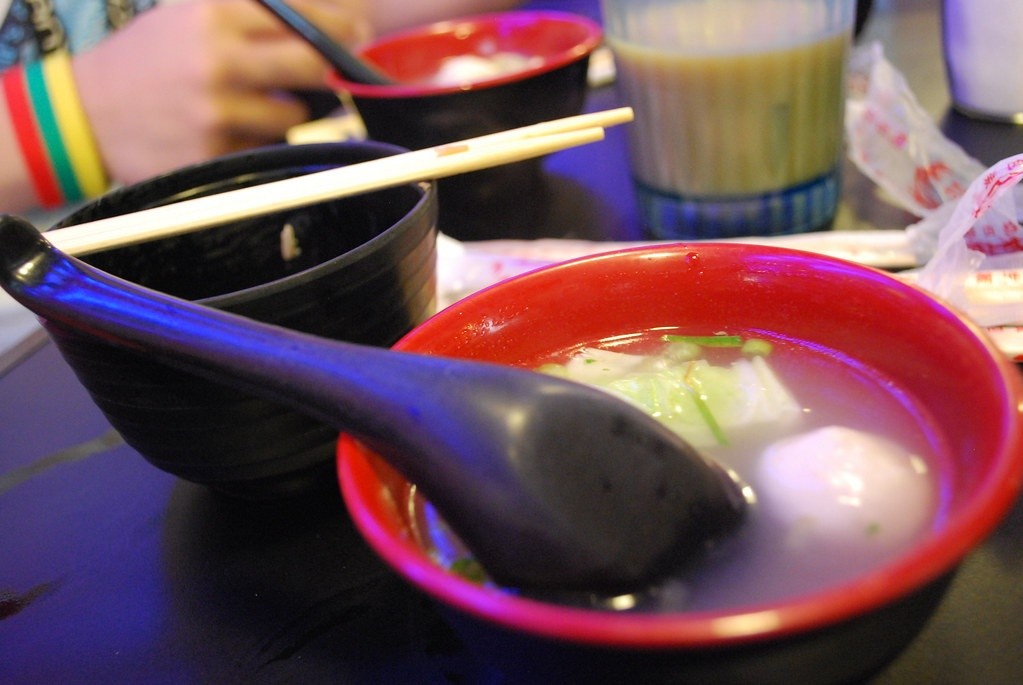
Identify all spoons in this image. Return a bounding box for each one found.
[0,216,747,588]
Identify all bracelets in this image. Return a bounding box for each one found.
[1,48,107,213]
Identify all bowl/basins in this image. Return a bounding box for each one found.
[337,241,1023,684]
[333,14,600,180]
[33,141,441,505]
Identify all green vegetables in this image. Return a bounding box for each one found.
[451,333,771,583]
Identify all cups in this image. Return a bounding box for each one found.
[939,0,1023,126]
[600,1,857,234]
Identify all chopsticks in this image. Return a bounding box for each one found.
[288,52,612,146]
[41,107,635,254]
[497,231,1023,359]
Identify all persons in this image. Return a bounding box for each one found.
[0,0,519,222]
